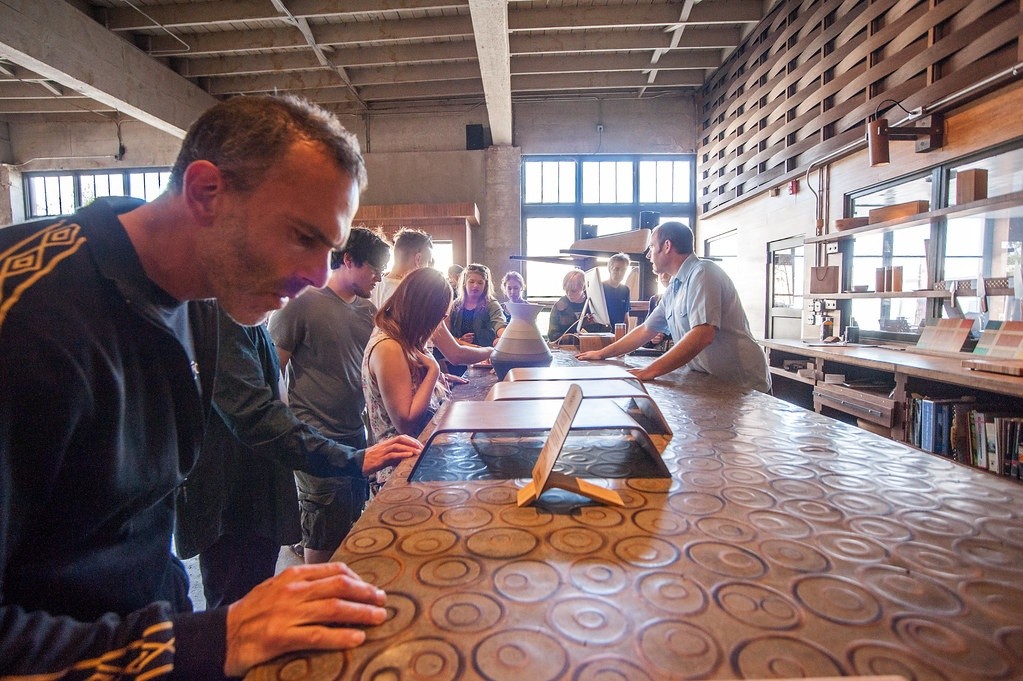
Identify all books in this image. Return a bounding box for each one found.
[903,390,1022,482]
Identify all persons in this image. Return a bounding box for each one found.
[368,228,495,386]
[571,220,774,397]
[0,88,388,681]
[499,271,533,324]
[176,300,425,609]
[427,258,509,385]
[360,267,452,496]
[643,267,672,349]
[599,253,630,337]
[268,227,390,561]
[547,268,602,345]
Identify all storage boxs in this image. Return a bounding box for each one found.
[956,169,988,205]
[869,199,930,227]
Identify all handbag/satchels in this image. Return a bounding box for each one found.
[810,266,839,294]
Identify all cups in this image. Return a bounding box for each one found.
[875,268,884,292]
[846,326,860,343]
[615,324,626,342]
[884,266,892,292]
[892,266,903,292]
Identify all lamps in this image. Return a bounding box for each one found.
[865,98,944,167]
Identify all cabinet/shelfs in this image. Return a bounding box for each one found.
[803,190,1023,299]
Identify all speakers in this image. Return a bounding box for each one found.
[639,211,660,230]
[580,224,597,240]
[466,124,484,150]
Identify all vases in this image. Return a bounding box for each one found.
[491,302,554,382]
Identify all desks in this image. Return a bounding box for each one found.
[756,337,1023,441]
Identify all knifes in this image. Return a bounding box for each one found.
[977,273,988,312]
[949,281,956,308]
[1014,260,1023,321]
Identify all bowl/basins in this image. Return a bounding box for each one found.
[853,286,868,291]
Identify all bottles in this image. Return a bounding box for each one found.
[822,317,834,340]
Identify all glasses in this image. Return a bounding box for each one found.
[417,233,432,252]
[443,314,448,320]
[469,264,485,271]
[364,262,384,277]
